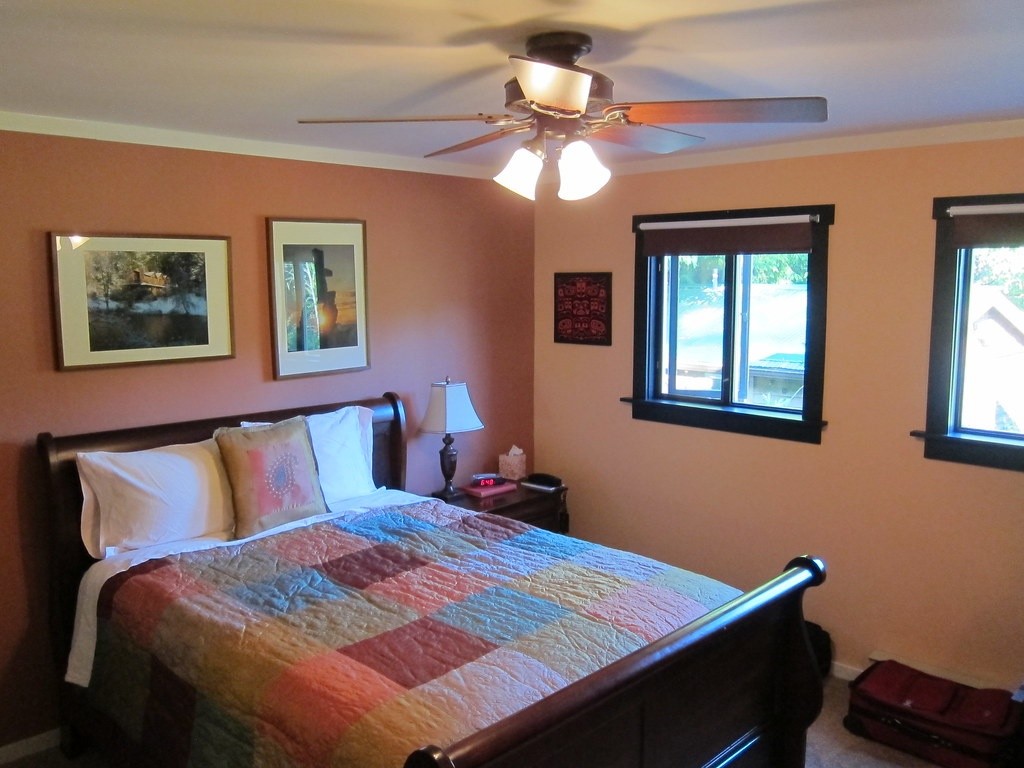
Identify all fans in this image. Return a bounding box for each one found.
[298,29,829,154]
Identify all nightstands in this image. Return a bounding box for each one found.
[425,481,569,537]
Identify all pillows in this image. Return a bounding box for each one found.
[240,405,388,517]
[78,433,237,557]
[214,415,332,541]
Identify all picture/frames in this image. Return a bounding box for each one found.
[49,230,237,373]
[554,272,613,345]
[267,217,372,383]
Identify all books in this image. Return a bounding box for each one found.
[459,482,517,497]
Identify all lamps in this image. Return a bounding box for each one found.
[490,33,611,203]
[416,377,485,504]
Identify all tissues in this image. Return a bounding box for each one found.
[499,444,527,481]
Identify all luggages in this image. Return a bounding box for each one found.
[843,658,1024,768]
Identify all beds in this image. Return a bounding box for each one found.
[33,391,828,767]
